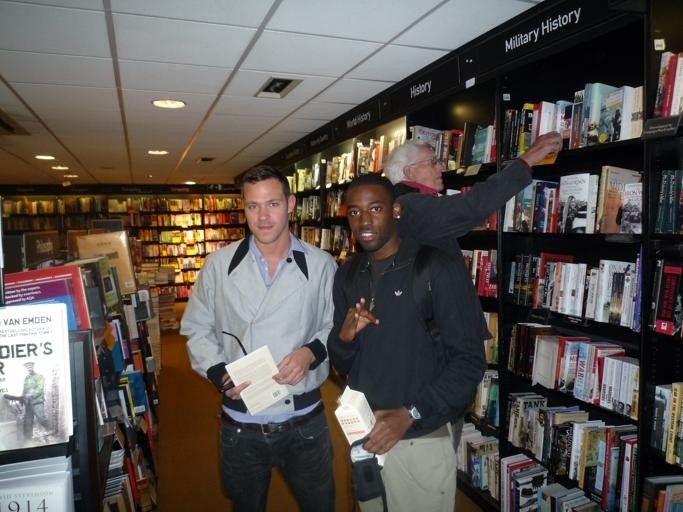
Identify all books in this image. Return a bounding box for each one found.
[642,51,683,510]
[159,192,249,335]
[460,248,639,420]
[278,124,452,267]
[453,83,642,233]
[456,371,637,511]
[0,194,158,511]
[224,344,291,417]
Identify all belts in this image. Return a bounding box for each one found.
[419,423,451,437]
[222,403,324,432]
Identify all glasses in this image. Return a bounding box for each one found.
[409,156,443,169]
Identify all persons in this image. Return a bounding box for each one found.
[324,170,486,510]
[382,129,564,458]
[176,163,339,512]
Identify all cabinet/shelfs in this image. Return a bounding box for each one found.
[233,0,682,511]
[1,181,233,512]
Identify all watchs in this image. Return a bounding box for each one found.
[402,399,422,424]
